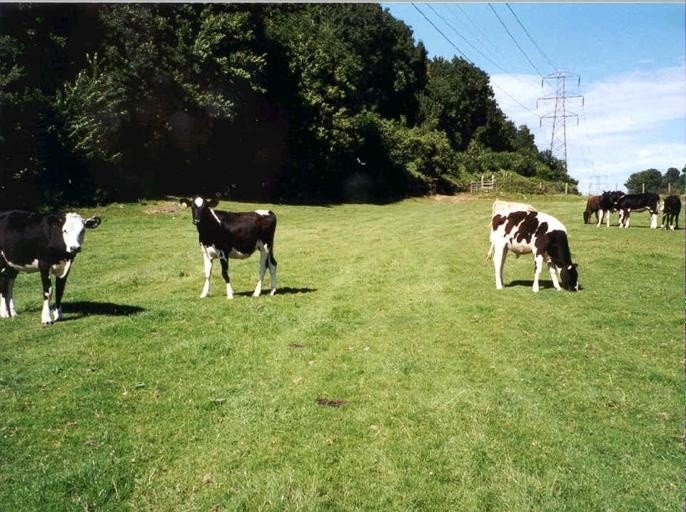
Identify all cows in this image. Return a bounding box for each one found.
[179,194,277,300]
[0,209,101,324]
[486,200,579,292]
[584,190,681,230]
